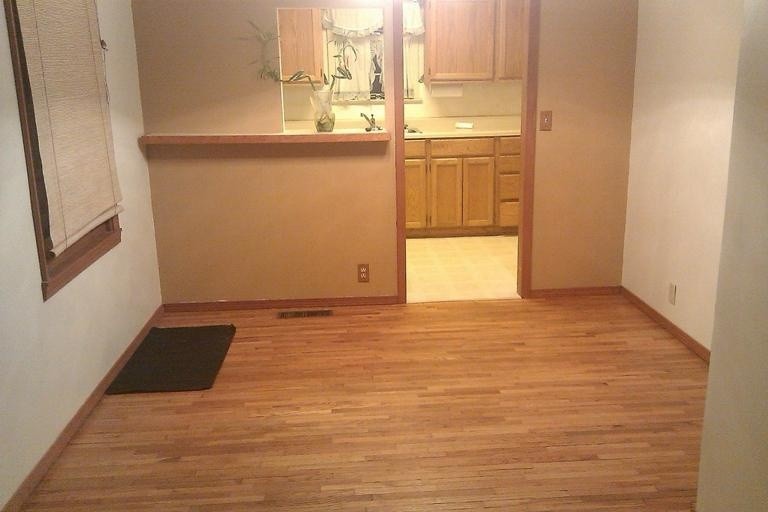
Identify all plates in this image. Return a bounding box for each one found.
[405,135,522,240]
[423,0,530,86]
[276,7,325,87]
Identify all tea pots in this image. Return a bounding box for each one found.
[320,0,424,104]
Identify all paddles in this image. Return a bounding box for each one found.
[104,325,236,395]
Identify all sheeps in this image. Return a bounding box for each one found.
[360,113,376,128]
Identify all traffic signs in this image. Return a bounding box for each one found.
[357,263,369,283]
[667,283,676,305]
[540,110,552,131]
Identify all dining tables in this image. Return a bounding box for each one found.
[232,18,360,132]
